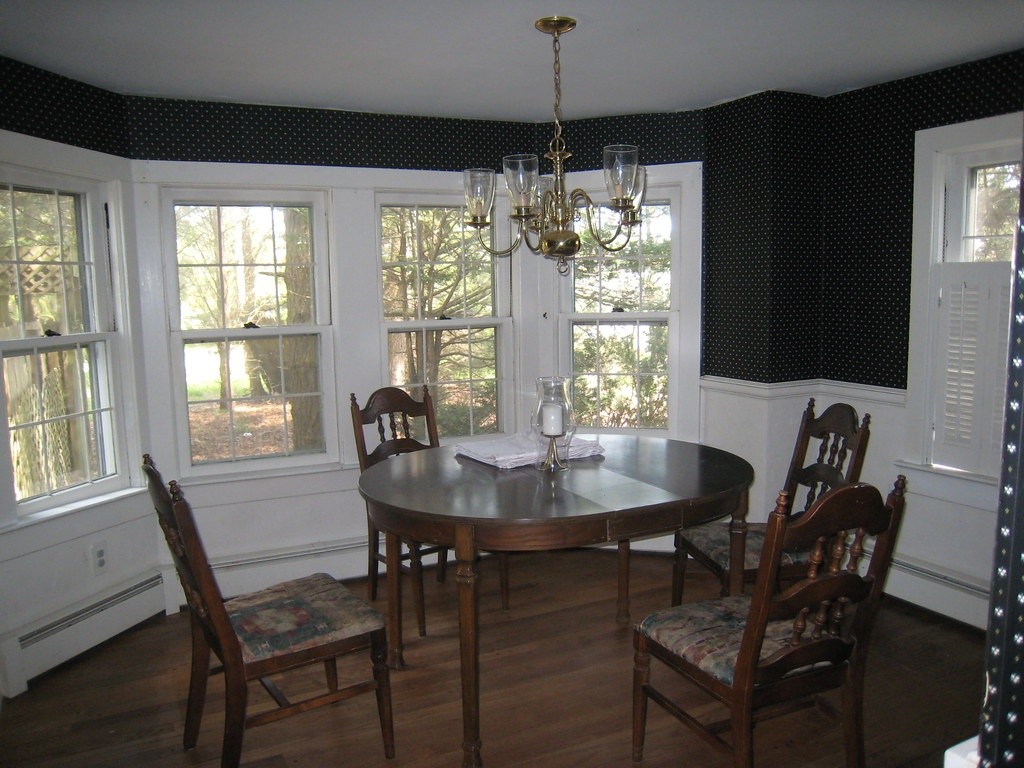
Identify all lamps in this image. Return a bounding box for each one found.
[462,15,646,273]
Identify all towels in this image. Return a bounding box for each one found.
[454,430,606,470]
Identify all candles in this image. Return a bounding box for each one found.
[530,376,577,472]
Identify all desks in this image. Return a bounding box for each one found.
[356,435,754,767]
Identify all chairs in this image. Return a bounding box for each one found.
[671,396,870,608]
[631,474,907,768]
[140,452,397,768]
[349,384,510,637]
